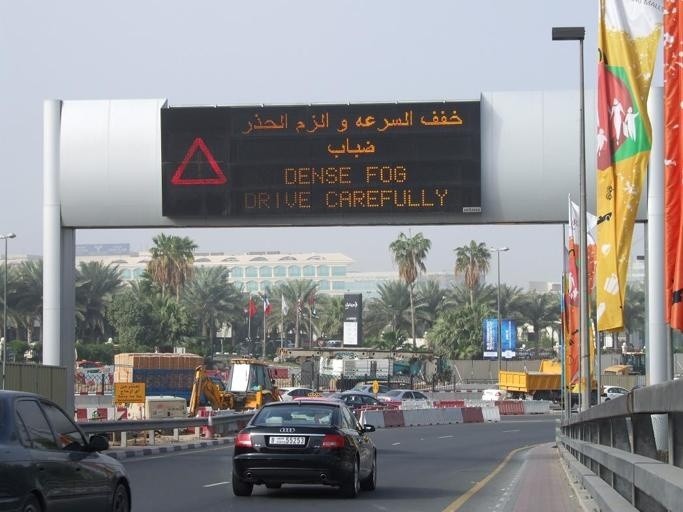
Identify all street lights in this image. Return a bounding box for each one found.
[0,232,16,391]
[491,246,509,372]
[550,26,590,411]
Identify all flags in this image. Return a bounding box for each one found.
[249,297,257,320]
[281,294,289,316]
[263,295,272,317]
[297,298,305,319]
[310,305,319,319]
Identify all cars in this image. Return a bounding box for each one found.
[601,386,631,403]
[482,383,518,401]
[280,380,428,405]
[0,391,131,512]
[233,400,376,498]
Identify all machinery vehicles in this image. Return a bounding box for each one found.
[188,360,284,417]
[604,350,645,377]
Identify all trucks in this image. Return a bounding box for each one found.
[497,358,597,409]
[114,368,225,407]
